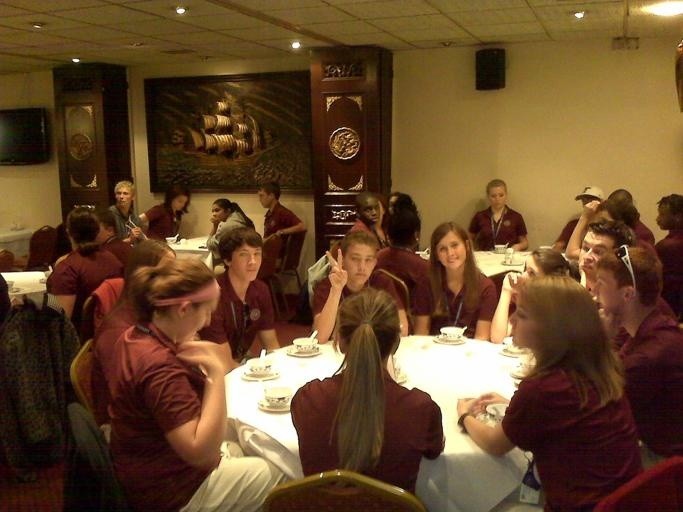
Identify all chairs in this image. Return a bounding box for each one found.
[2,219,682,511]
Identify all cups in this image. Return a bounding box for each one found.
[504,338,526,353]
[6,280,13,290]
[440,326,464,340]
[165,237,178,244]
[486,402,510,425]
[494,244,514,264]
[263,388,292,408]
[44,270,51,278]
[246,358,273,376]
[518,355,537,377]
[293,337,318,353]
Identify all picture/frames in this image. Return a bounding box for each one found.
[142,67,318,197]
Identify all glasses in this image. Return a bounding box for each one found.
[615,244,636,287]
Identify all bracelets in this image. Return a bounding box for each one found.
[458,412,474,429]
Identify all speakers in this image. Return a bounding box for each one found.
[475,48,506,91]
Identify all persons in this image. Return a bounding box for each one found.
[198,228,281,369]
[107,258,289,512]
[312,192,498,345]
[258,182,307,269]
[206,199,255,275]
[96,212,132,279]
[467,180,530,251]
[590,247,683,471]
[489,186,683,344]
[46,208,124,336]
[107,180,141,246]
[139,185,190,243]
[457,274,645,512]
[290,288,445,496]
[91,240,211,416]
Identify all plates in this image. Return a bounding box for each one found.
[500,348,524,358]
[7,287,16,293]
[259,398,293,411]
[242,373,280,382]
[508,366,529,382]
[38,278,46,283]
[432,334,467,345]
[286,347,323,356]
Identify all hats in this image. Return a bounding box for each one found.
[574,186,604,201]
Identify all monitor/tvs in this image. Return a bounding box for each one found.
[0,106,50,165]
